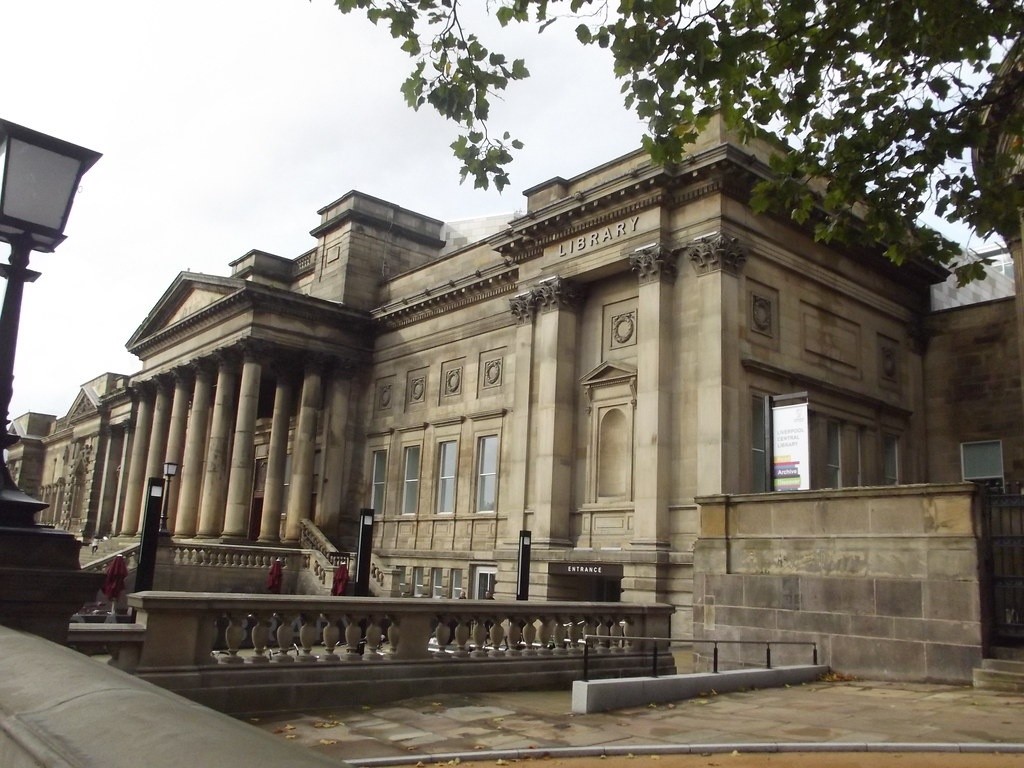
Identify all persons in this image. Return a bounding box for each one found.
[92,535,99,555]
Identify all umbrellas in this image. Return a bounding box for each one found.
[100,554,128,616]
[331,562,349,595]
[267,557,282,593]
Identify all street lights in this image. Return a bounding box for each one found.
[151,463,179,590]
[0,115,108,652]
